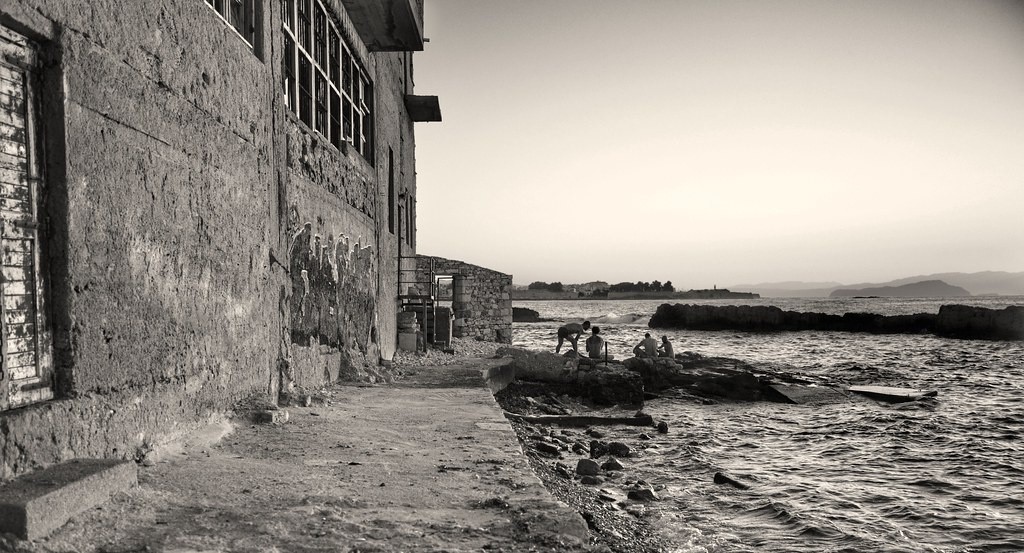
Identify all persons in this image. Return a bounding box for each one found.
[586,326,604,359]
[556,321,590,358]
[657,336,675,359]
[633,333,658,358]
